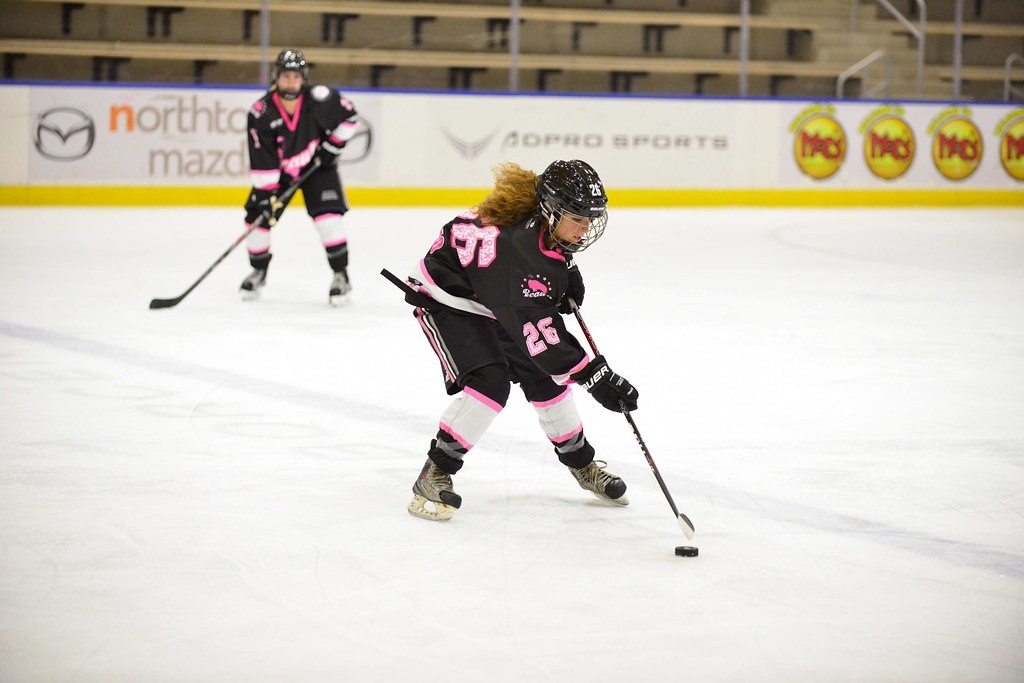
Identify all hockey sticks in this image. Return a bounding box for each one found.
[148,153,323,311]
[562,296,699,538]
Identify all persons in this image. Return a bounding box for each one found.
[240,48,357,305]
[405,158,639,519]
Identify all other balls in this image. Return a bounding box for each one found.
[674,544,700,557]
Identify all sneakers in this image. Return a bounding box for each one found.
[407,439,465,520]
[554,446,629,505]
[238,267,267,296]
[329,269,352,305]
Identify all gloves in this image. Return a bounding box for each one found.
[252,191,283,225]
[571,354,639,414]
[562,267,586,314]
[309,136,347,167]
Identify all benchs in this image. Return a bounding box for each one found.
[1,0,863,102]
[881,17,1024,100]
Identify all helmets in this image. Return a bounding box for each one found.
[537,159,608,252]
[275,50,309,97]
